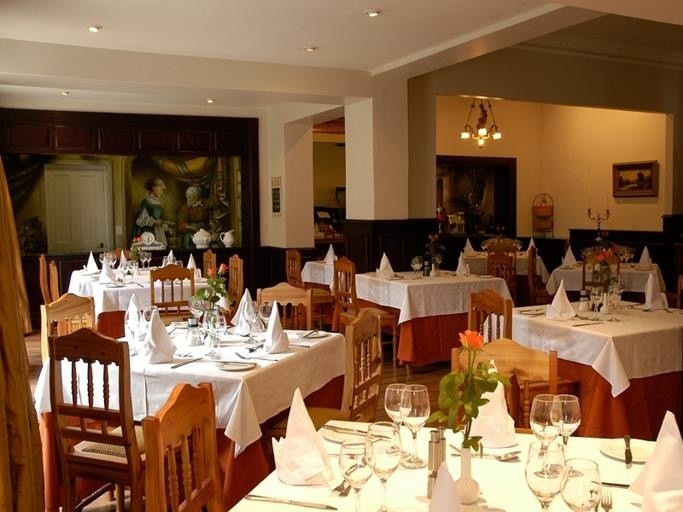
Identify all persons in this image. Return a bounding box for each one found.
[178,187,210,250]
[132,177,176,247]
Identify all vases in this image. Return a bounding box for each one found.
[430,263,439,277]
[600,293,609,314]
[203,303,226,339]
[454,447,481,505]
[131,260,139,276]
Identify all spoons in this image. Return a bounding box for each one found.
[450,450,522,461]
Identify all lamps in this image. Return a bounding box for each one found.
[587,191,610,241]
[461,99,502,147]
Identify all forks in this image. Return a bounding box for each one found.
[449,443,489,456]
[663,307,681,313]
[600,487,612,512]
[235,352,278,361]
[330,462,359,492]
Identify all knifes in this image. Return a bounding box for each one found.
[170,357,202,368]
[572,322,605,327]
[624,434,633,466]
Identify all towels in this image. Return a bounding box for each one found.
[119,249,126,265]
[271,386,330,487]
[638,245,652,268]
[526,236,538,259]
[147,309,176,364]
[431,460,462,511]
[325,243,335,265]
[265,300,290,355]
[644,273,665,312]
[547,280,577,322]
[84,250,100,277]
[100,256,117,284]
[186,253,198,282]
[464,238,477,257]
[167,249,174,264]
[637,411,683,512]
[231,288,266,334]
[125,293,147,343]
[456,252,468,276]
[468,359,517,450]
[379,252,395,279]
[560,245,577,270]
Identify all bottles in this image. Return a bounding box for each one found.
[423,243,430,276]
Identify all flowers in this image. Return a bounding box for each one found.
[424,234,446,263]
[591,251,618,293]
[425,326,511,449]
[188,263,230,305]
[130,236,141,261]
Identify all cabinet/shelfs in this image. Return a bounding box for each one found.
[532,192,554,239]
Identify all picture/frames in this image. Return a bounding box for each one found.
[612,160,658,197]
[121,154,241,254]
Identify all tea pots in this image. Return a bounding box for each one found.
[219,229,235,247]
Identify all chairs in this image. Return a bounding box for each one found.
[143,383,222,512]
[269,310,381,441]
[145,265,194,326]
[581,255,619,293]
[256,282,312,330]
[470,288,511,340]
[333,257,395,370]
[286,250,334,330]
[527,246,552,305]
[47,327,145,511]
[228,255,243,309]
[452,339,558,427]
[486,250,516,298]
[203,250,216,278]
[38,255,88,324]
[40,293,95,361]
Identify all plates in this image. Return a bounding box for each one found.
[169,323,188,329]
[519,309,545,315]
[633,267,652,271]
[295,330,329,338]
[598,437,655,464]
[215,361,256,372]
[475,274,493,278]
[320,424,381,443]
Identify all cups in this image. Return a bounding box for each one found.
[99,253,105,271]
[202,309,221,357]
[399,383,431,470]
[607,283,623,323]
[251,301,258,314]
[128,261,137,280]
[142,305,157,323]
[558,457,604,512]
[110,255,118,270]
[522,439,571,511]
[383,383,413,457]
[188,295,205,331]
[145,252,152,270]
[413,255,424,280]
[119,262,127,279]
[589,286,604,322]
[139,251,147,271]
[548,394,582,481]
[174,260,183,269]
[410,257,416,280]
[365,421,403,512]
[338,437,377,511]
[123,309,143,356]
[209,316,227,359]
[258,303,272,329]
[529,393,564,480]
